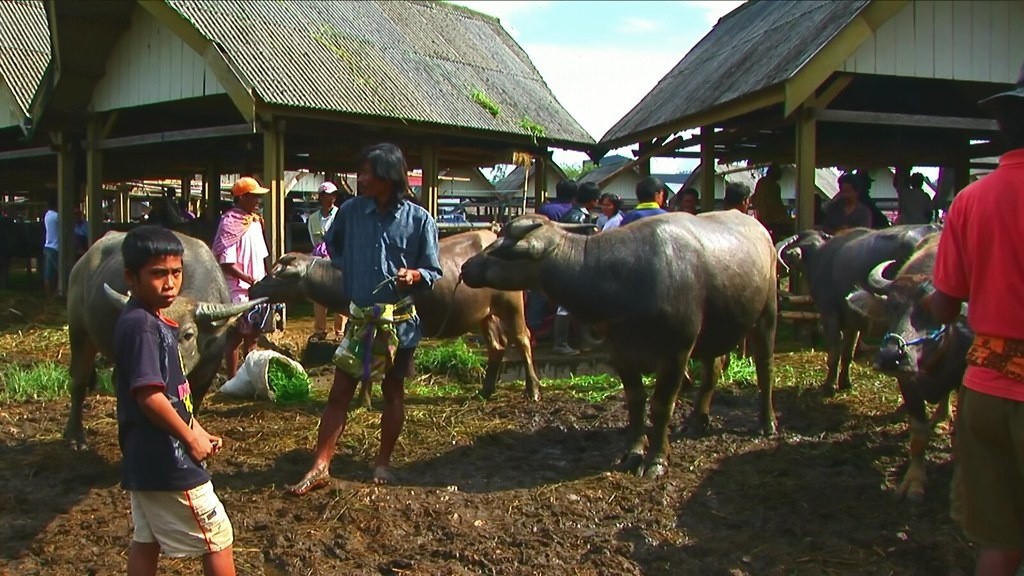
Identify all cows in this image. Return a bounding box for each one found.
[845,231,974,519]
[773,223,944,395]
[246,228,542,409]
[60,226,271,451]
[461,207,779,480]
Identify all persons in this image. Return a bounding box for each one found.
[915,85,1024,576]
[528,169,933,360]
[39,143,443,576]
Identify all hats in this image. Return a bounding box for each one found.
[318,182,339,194]
[980,59,1024,122]
[232,176,270,197]
